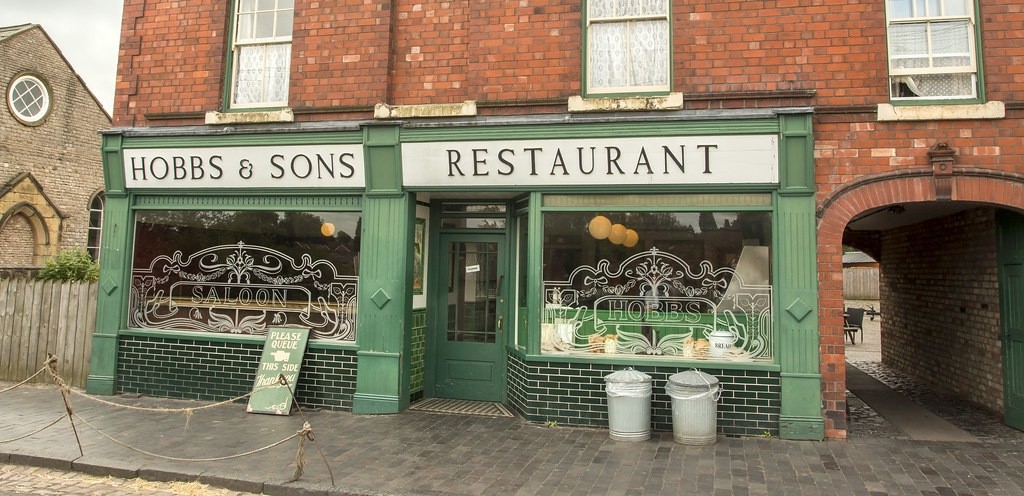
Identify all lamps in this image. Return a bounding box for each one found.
[622,212,639,248]
[589,212,612,240]
[608,212,627,245]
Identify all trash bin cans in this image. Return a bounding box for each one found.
[668,371,719,445]
[603,368,652,442]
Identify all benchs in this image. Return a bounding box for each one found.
[844,328,858,345]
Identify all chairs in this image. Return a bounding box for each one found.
[844,307,864,343]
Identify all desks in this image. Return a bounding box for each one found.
[844,314,850,328]
